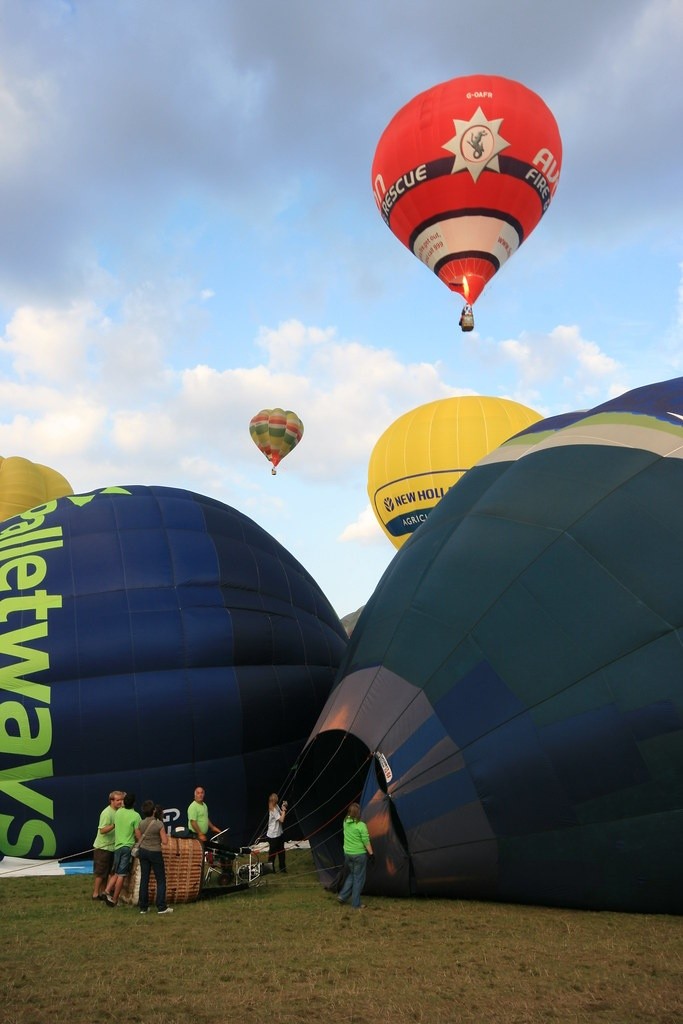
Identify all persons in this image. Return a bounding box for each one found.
[138,800,174,914]
[91,791,142,907]
[337,803,375,909]
[266,793,288,875]
[187,786,221,842]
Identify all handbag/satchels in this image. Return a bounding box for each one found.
[130,843,140,856]
[171,826,197,839]
[204,860,222,886]
[238,862,264,879]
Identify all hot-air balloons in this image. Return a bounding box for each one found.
[249,408,305,475]
[0,487,350,860]
[368,395,543,554]
[105,375,683,915]
[371,73,563,335]
[0,453,79,526]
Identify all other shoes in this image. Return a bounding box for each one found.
[140,907,150,914]
[105,895,117,907]
[158,908,173,913]
[272,869,276,874]
[92,894,101,900]
[358,903,367,908]
[337,898,345,905]
[102,890,110,900]
[280,868,287,874]
